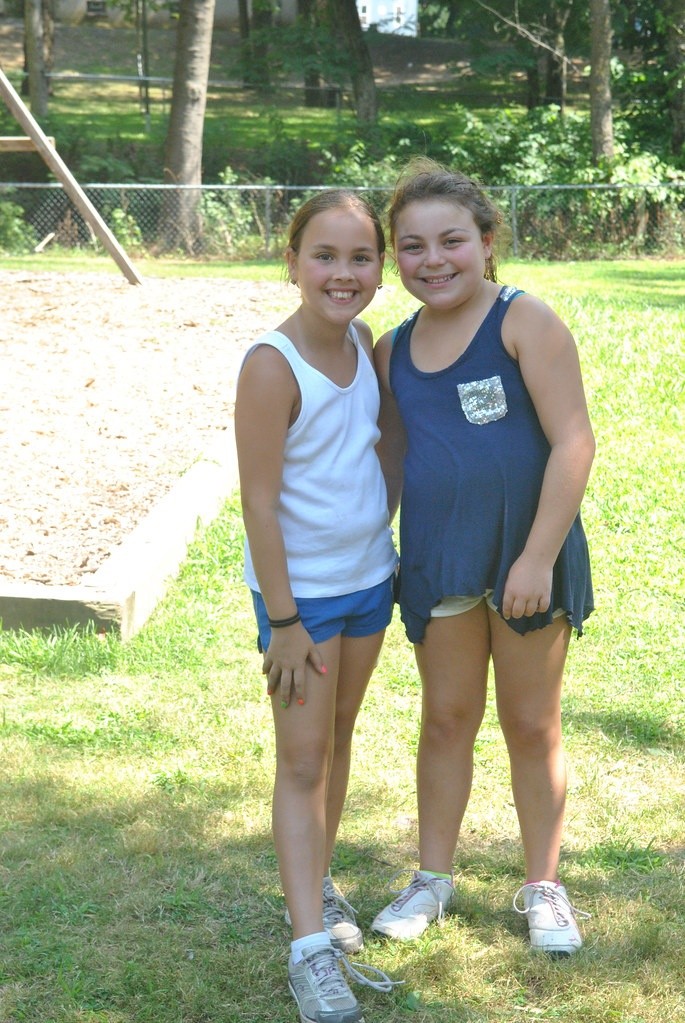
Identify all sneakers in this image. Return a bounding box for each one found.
[286,943,405,1023]
[369,869,455,942]
[513,880,591,958]
[285,876,364,954]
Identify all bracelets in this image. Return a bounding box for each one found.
[266,610,301,628]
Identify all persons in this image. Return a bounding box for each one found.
[232,191,404,1023]
[374,158,597,957]
